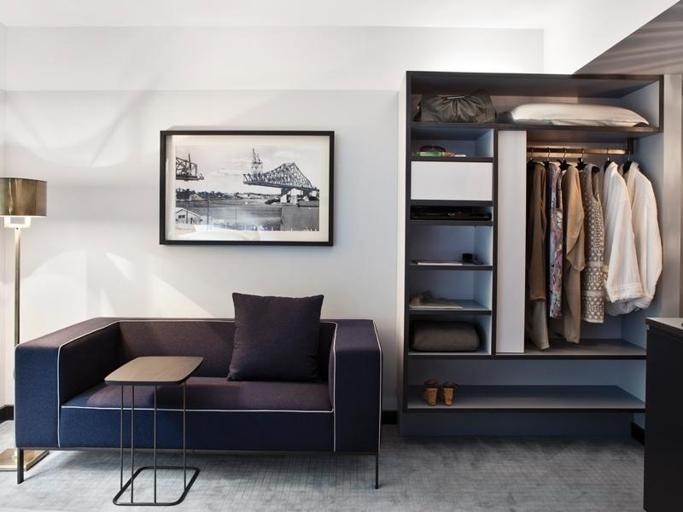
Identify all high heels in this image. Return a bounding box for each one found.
[422,379,455,405]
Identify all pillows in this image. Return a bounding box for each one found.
[499,103,651,128]
[227,293,324,384]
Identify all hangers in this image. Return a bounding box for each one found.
[529,140,635,174]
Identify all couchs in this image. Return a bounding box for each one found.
[16,317,383,489]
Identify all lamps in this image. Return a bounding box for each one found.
[0,177,49,472]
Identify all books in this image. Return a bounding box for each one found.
[410,151,455,158]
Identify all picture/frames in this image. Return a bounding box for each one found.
[160,131,334,246]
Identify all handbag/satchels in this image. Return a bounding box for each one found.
[413,89,496,122]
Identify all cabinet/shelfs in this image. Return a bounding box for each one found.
[398,70,683,142]
[398,123,499,356]
[395,357,648,443]
[527,123,683,361]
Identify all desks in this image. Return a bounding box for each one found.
[104,356,204,506]
[643,318,683,511]
